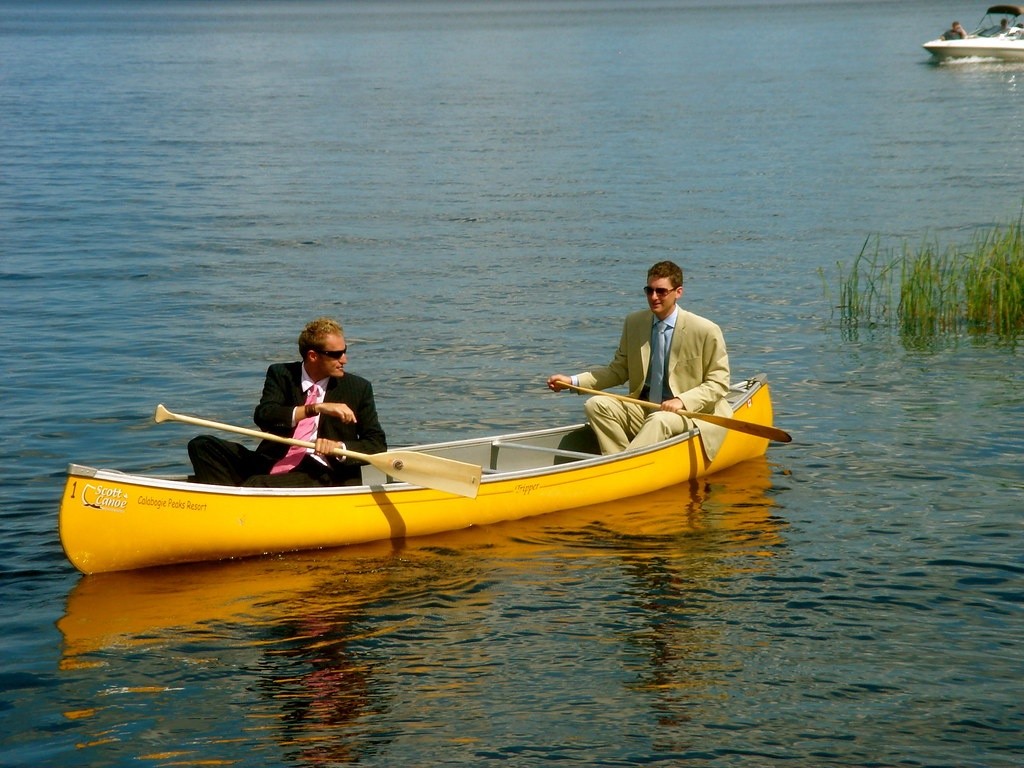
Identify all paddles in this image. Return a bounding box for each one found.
[553,378,793,444]
[153,403,484,501]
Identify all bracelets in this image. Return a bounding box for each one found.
[304,404,319,418]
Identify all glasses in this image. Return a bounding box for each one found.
[643,286,676,297]
[314,343,347,359]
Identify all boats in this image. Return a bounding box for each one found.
[922,5,1024,59]
[59,376,792,575]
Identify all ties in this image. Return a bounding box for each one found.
[649,321,668,403]
[270,384,318,474]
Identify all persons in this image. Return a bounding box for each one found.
[940,21,967,40]
[547,261,734,462]
[999,18,1011,29]
[188,317,388,488]
